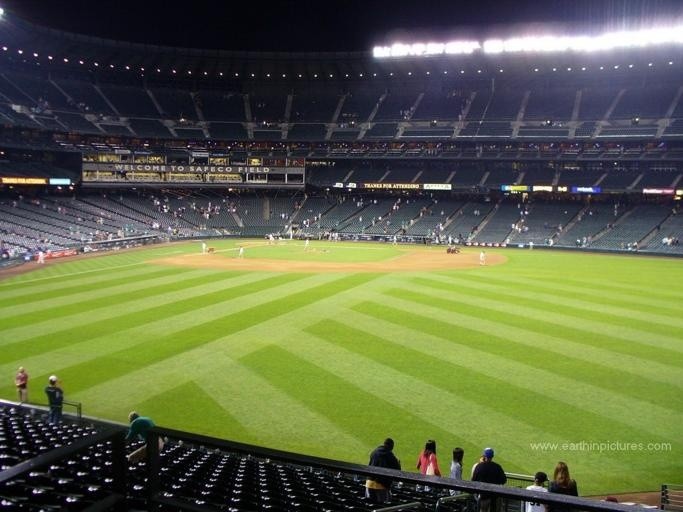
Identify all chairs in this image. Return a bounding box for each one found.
[1,400,664,512]
[200,71,680,258]
[1,74,201,270]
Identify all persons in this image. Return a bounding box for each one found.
[520,470,551,511]
[449,446,465,497]
[470,447,508,511]
[43,374,63,423]
[123,411,160,474]
[414,438,441,494]
[12,366,28,404]
[546,461,580,511]
[1,87,683,246]
[362,437,402,504]
[36,250,45,264]
[479,250,487,266]
[469,454,488,481]
[445,245,461,254]
[201,241,207,254]
[237,246,244,258]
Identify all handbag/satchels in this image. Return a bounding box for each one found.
[426,462,435,475]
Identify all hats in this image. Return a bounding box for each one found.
[484,448,493,457]
[535,472,548,482]
[49,375,57,382]
